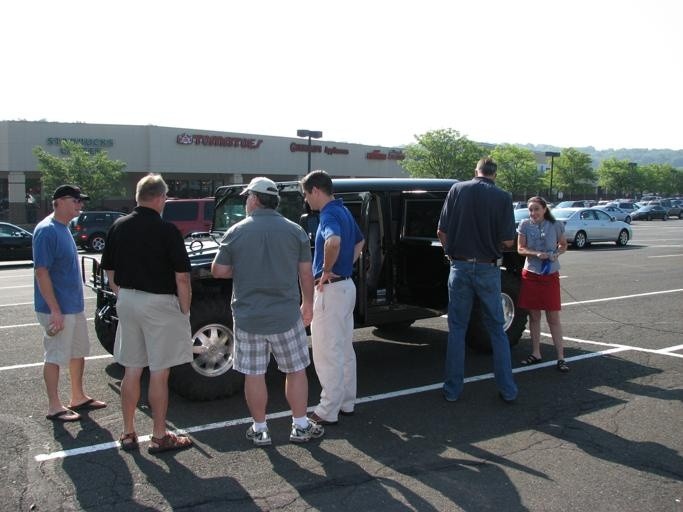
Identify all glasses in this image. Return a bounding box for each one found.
[73,199,81,203]
[540,228,545,238]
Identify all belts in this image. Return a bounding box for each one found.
[314,275,349,286]
[467,257,496,263]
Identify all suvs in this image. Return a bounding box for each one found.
[606,202,641,212]
[69,210,128,252]
[81,179,529,402]
[162,197,215,240]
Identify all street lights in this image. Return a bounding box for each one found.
[545,151,560,201]
[297,130,322,173]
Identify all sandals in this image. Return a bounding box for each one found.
[148,432,193,453]
[119,432,138,450]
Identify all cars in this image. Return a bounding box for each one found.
[0,221,33,261]
[593,205,631,224]
[631,205,669,221]
[552,207,632,248]
[511,209,529,228]
[555,201,585,207]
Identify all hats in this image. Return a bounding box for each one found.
[239,176,278,196]
[53,184,89,199]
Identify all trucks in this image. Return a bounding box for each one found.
[666,201,683,219]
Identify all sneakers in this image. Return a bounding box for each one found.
[289,417,324,442]
[245,425,272,446]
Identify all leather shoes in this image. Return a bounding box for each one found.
[308,413,336,424]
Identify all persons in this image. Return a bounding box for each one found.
[301,169,366,426]
[438,157,519,404]
[32,184,106,420]
[516,196,570,371]
[210,177,325,446]
[99,173,193,453]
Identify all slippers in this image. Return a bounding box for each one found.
[520,354,542,365]
[46,409,81,421]
[68,398,107,410]
[557,360,568,370]
